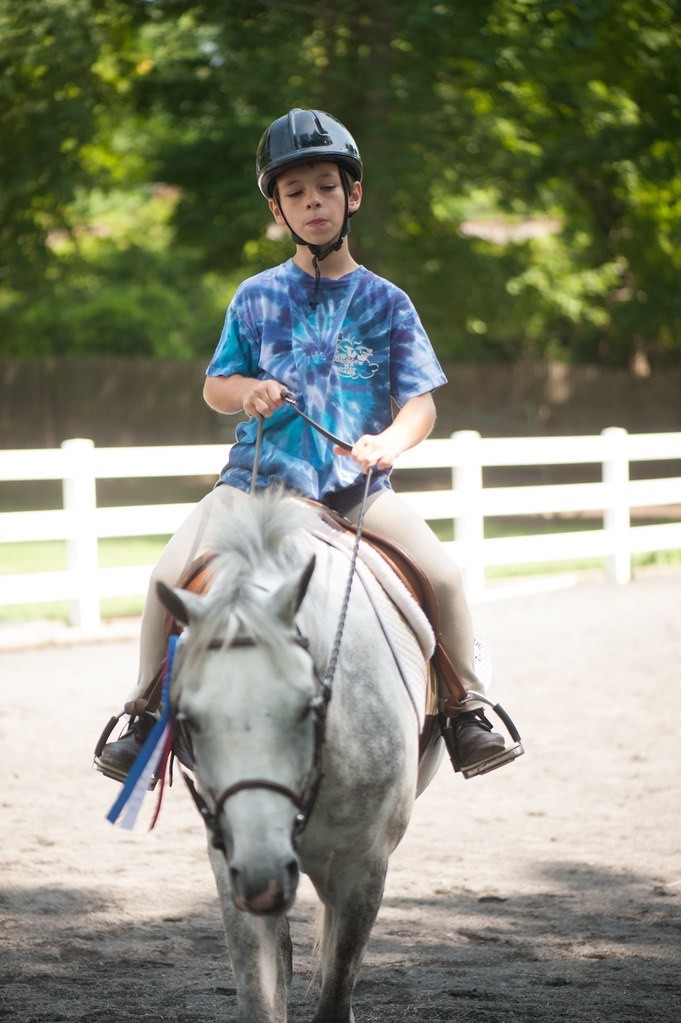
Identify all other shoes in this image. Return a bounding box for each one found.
[99,715,158,774]
[448,711,505,770]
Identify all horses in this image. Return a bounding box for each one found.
[157,494,443,1023]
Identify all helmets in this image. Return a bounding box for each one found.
[256,108,363,200]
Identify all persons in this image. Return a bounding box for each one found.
[99,108,506,792]
